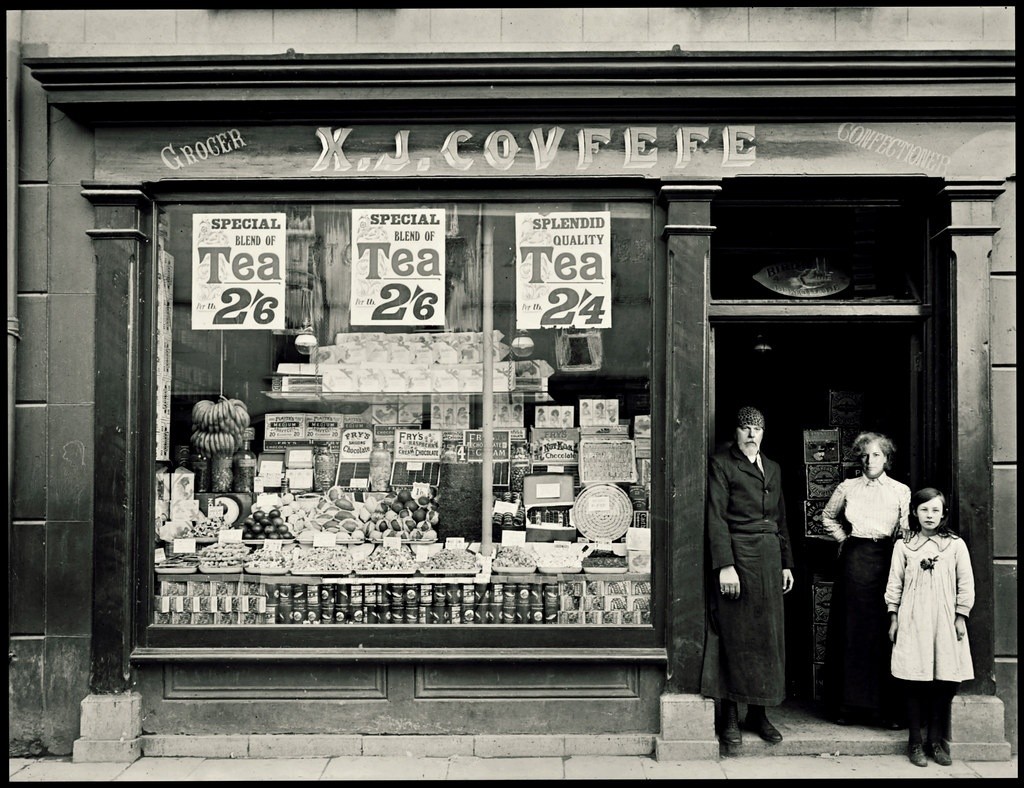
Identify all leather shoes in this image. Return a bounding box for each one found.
[722,707,743,745]
[745,712,784,741]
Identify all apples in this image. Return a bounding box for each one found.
[235,510,294,540]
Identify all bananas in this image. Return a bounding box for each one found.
[190,398,251,459]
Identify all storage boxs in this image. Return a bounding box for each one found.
[169,393,651,540]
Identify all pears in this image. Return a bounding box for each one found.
[364,489,438,540]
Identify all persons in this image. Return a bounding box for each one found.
[885,488,974,767]
[700,407,794,744]
[821,431,915,730]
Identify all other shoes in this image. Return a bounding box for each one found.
[909,744,928,767]
[926,742,952,766]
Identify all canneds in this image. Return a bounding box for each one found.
[264,584,558,624]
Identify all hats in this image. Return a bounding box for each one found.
[736,406,766,430]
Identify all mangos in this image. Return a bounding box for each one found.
[299,487,364,540]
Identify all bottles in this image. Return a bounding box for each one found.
[280,478,291,497]
[170,445,195,533]
[154,460,170,532]
